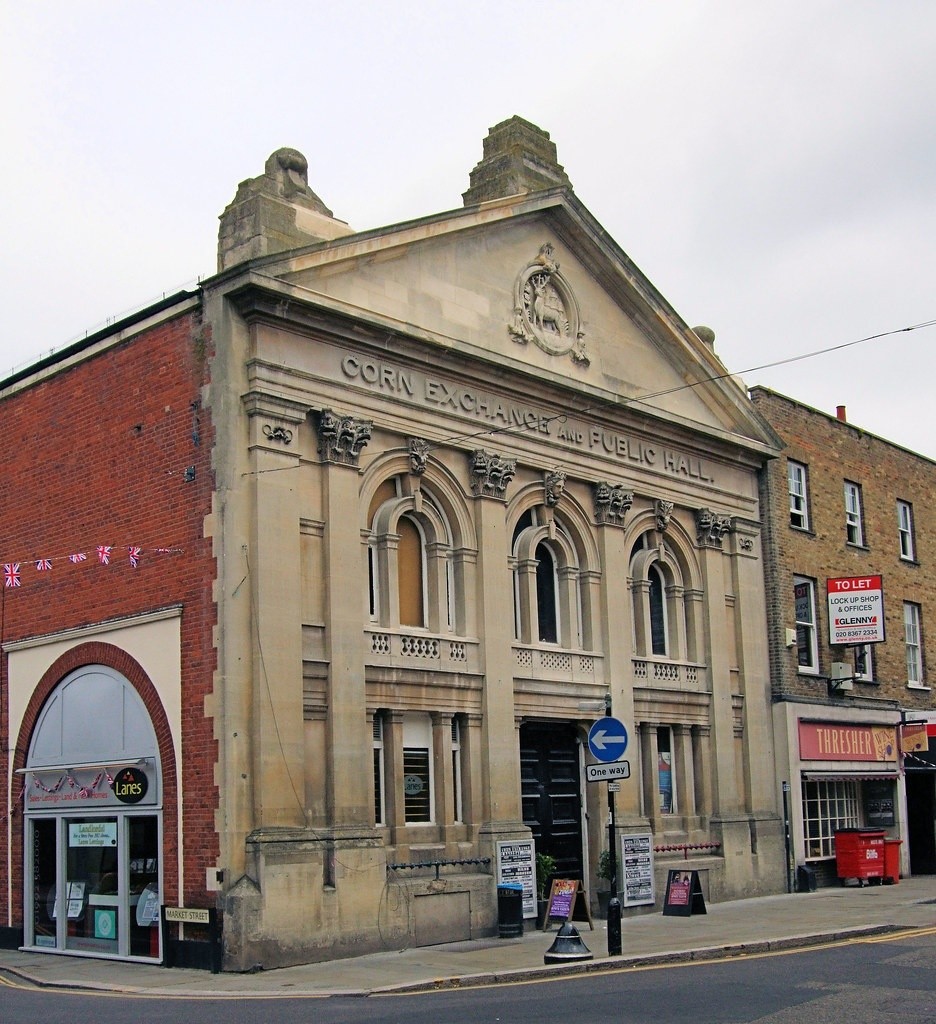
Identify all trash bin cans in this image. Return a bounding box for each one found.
[497,883,524,939]
[884,839,903,885]
[833,827,889,888]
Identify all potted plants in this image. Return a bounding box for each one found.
[596,848,626,919]
[535,853,557,931]
